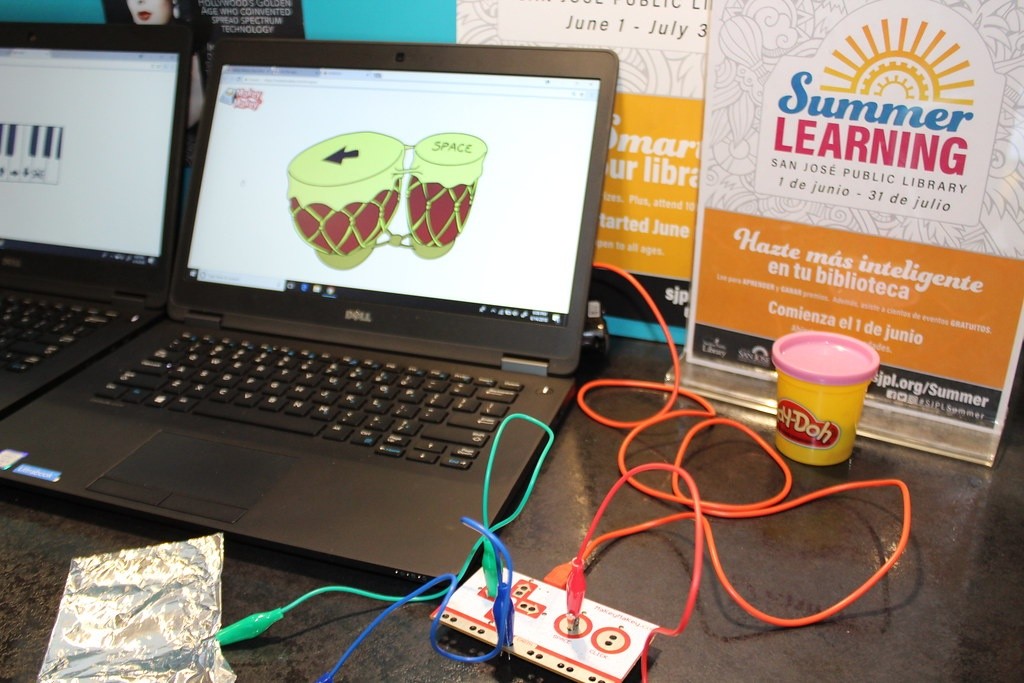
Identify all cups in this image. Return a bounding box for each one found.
[772,331,878,465]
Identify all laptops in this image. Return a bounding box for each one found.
[0,20,621,588]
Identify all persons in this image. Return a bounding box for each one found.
[127,0,205,166]
